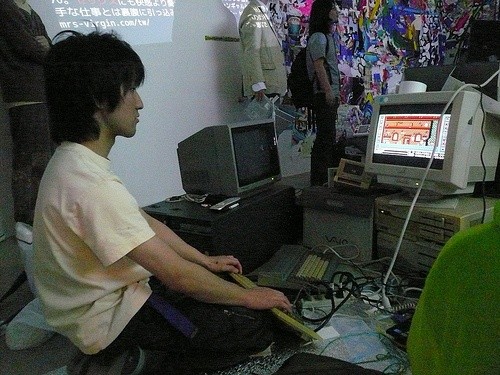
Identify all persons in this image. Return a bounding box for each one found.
[32,29,293,375]
[238,0,287,121]
[0,0,54,227]
[306,0,341,174]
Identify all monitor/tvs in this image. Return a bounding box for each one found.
[364,91,500,194]
[177,118,282,198]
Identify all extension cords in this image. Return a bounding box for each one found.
[302,291,351,308]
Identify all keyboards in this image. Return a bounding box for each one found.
[258,245,338,292]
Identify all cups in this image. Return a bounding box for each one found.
[391,81,427,93]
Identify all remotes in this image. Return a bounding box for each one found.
[210,198,240,211]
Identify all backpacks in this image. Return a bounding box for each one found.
[287,31,329,109]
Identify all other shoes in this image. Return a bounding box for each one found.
[67,341,156,375]
